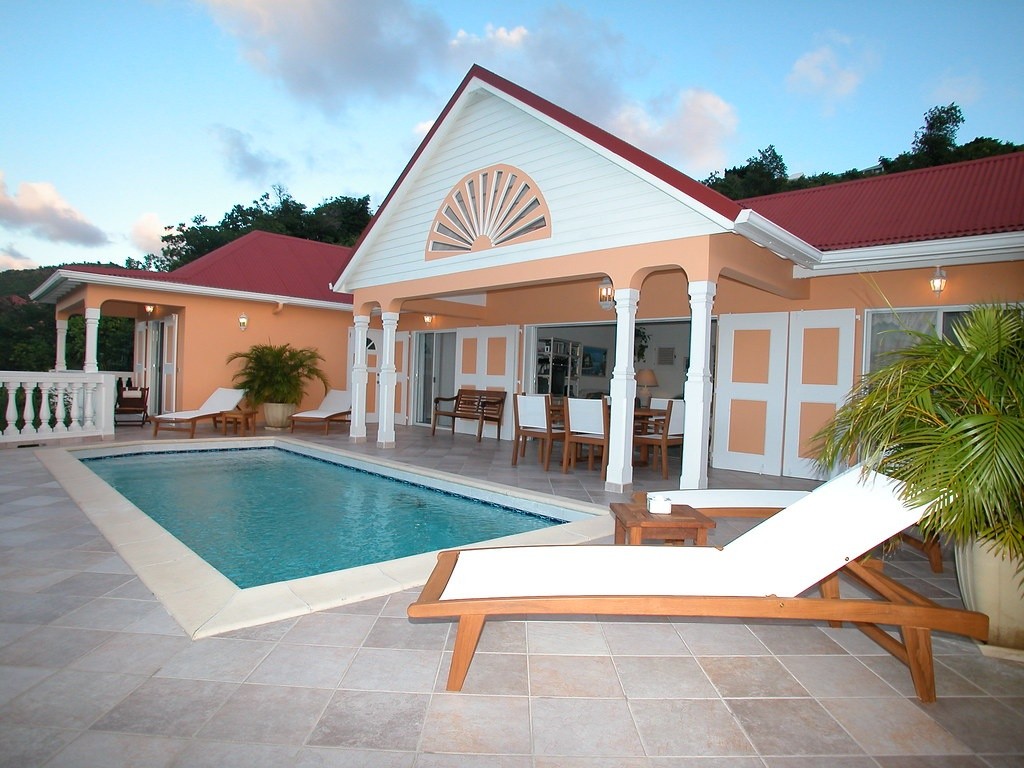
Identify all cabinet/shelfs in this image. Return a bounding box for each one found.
[536,337,582,399]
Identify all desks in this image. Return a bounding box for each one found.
[549,405,666,465]
[610,501,717,551]
[219,410,259,436]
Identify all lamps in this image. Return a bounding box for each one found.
[238,312,249,332]
[635,368,659,407]
[423,313,432,327]
[144,304,154,315]
[599,276,615,310]
[930,265,947,298]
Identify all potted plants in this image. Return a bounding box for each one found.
[805,276,1024,651]
[227,341,330,433]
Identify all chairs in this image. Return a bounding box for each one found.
[632,443,946,573]
[114,387,151,428]
[287,389,352,435]
[406,452,991,703]
[150,387,250,439]
[432,389,507,443]
[512,391,686,480]
